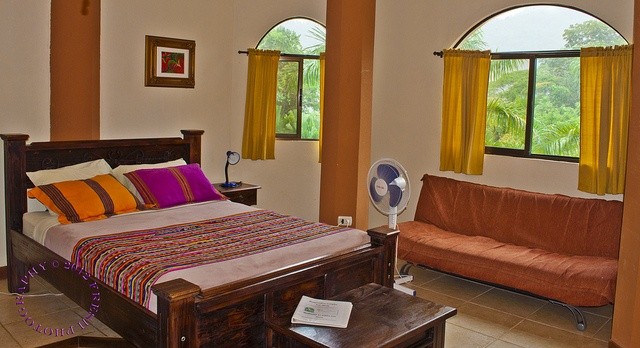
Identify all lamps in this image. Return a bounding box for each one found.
[219,149,240,188]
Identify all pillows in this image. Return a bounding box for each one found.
[26,158,113,217]
[109,158,186,206]
[28,174,156,225]
[123,163,229,209]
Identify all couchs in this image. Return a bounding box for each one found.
[397,174,624,331]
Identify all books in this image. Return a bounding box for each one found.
[291,295,353,329]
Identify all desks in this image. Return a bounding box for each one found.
[266,283,457,348]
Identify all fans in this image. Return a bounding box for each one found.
[367,157,417,296]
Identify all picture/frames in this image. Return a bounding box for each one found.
[144,34,196,89]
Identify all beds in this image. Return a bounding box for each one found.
[0,128,400,348]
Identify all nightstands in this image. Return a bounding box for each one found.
[210,182,262,206]
